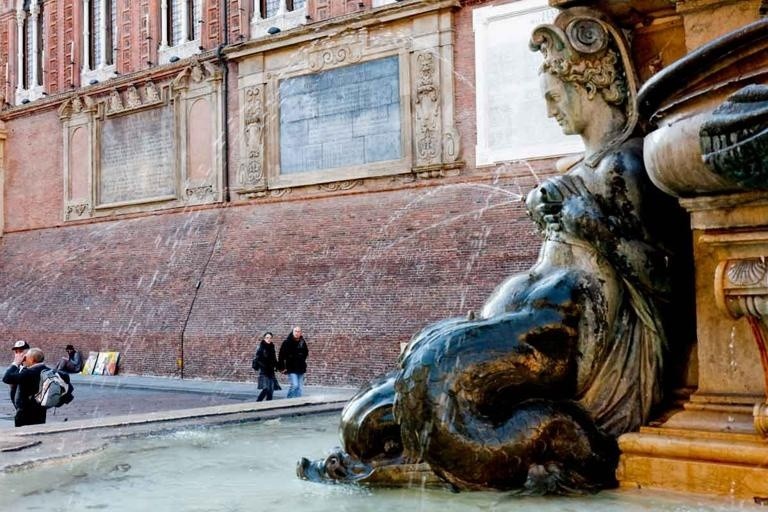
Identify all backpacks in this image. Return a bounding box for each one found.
[31,366,74,406]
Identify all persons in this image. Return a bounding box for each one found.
[2,347,46,427]
[254,332,281,402]
[10,340,30,406]
[55,344,82,373]
[340,5,692,499]
[278,326,309,398]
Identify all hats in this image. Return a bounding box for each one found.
[11,340,29,350]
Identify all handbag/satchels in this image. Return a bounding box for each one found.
[252,358,259,371]
